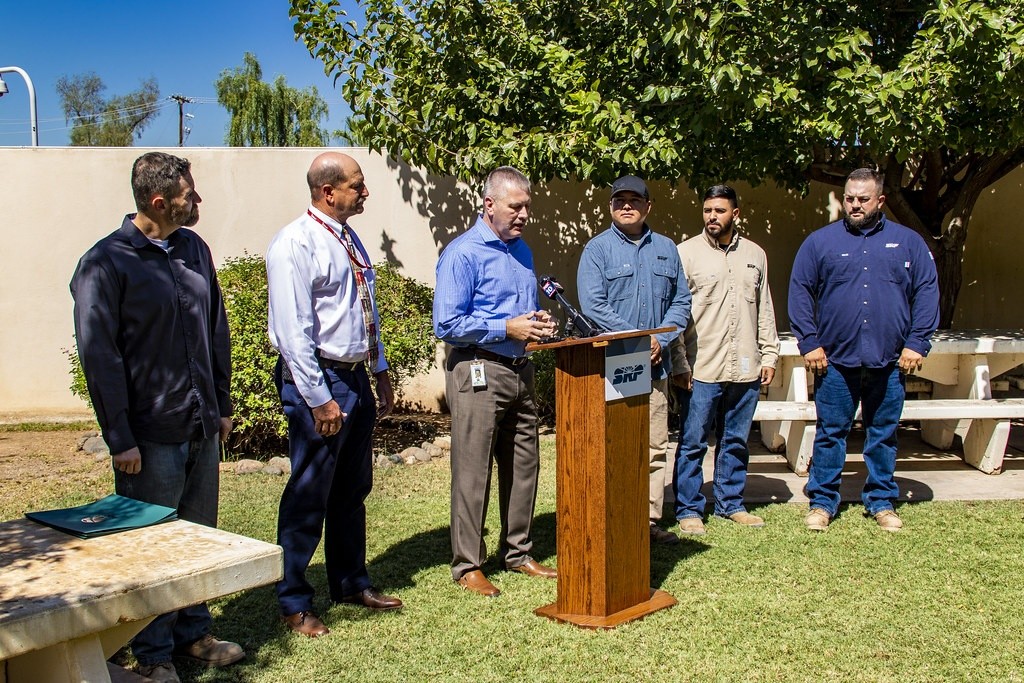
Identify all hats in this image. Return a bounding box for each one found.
[610,175,649,202]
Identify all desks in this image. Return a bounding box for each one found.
[759,329,1024,476]
[0,520,284,683]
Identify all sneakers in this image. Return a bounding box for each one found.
[138,660,179,683]
[873,510,903,532]
[650,521,680,546]
[728,510,765,526]
[679,518,707,535]
[803,507,830,531]
[172,633,246,667]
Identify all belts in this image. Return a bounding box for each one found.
[317,357,364,372]
[454,345,528,367]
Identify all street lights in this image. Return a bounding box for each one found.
[0,66,39,147]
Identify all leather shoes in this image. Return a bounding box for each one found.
[506,558,557,580]
[330,588,403,610]
[455,569,501,596]
[284,610,330,638]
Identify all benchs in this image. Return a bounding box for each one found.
[760,380,1009,394]
[753,398,1024,421]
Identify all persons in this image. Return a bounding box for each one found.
[431,168,557,597]
[578,175,692,541]
[669,185,781,533]
[70,153,244,683]
[265,153,403,637]
[788,169,940,532]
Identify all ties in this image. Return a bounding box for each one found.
[342,228,380,370]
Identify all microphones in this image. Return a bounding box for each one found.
[538,273,599,338]
